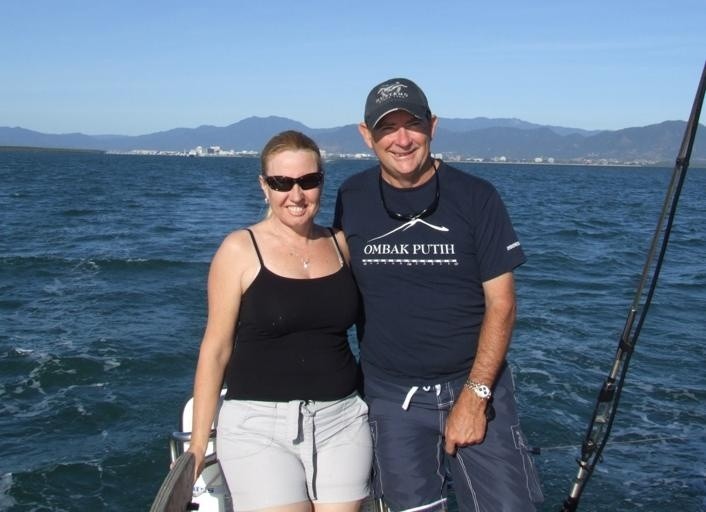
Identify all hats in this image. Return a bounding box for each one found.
[365,78,431,130]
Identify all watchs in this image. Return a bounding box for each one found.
[464,379,492,400]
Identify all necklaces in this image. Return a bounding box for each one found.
[279,234,311,270]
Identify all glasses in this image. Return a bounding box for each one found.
[261,172,324,191]
[378,161,441,221]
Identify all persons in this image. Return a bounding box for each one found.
[333,77,546,510]
[168,130,376,512]
